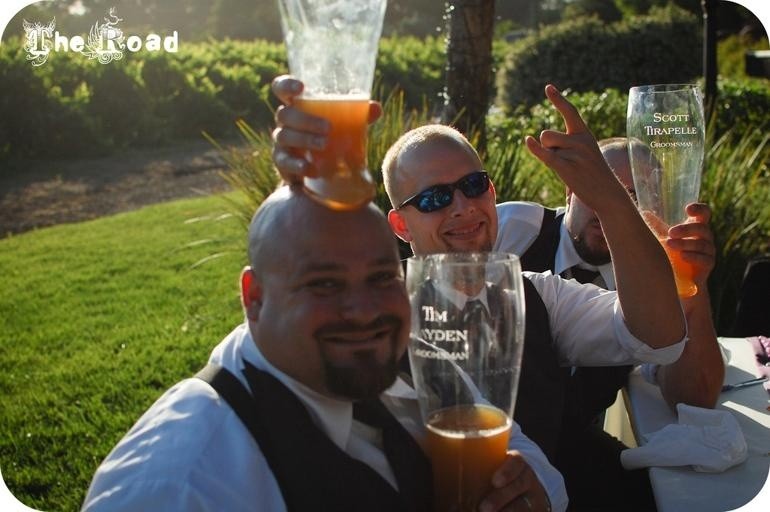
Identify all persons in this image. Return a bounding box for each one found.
[77,180,573,512]
[492,134,726,511]
[270,74,693,510]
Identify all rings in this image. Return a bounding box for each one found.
[523,492,533,511]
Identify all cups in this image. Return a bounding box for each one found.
[405,252,528,501]
[271,0,386,210]
[629,83,707,298]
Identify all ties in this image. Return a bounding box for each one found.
[350,395,437,511]
[570,264,602,285]
[463,298,484,393]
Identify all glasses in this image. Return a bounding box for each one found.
[394,167,491,215]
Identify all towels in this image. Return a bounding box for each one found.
[620,402,749,474]
